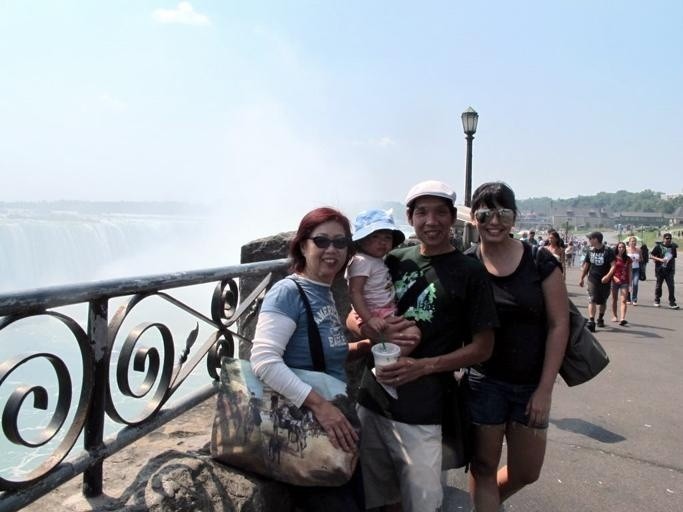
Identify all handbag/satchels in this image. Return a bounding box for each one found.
[559,298,612,387]
[208,357,362,486]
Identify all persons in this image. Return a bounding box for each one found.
[344,206,422,401]
[460,182,570,512]
[249,207,373,512]
[520,229,679,333]
[345,180,501,512]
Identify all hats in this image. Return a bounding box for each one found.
[404,180,456,207]
[349,208,404,249]
[585,231,602,242]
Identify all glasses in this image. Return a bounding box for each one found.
[304,237,350,249]
[473,209,516,227]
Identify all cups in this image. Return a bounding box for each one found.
[661,252,672,268]
[370,342,400,384]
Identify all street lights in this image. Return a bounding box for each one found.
[460,105,479,208]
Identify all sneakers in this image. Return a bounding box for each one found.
[586,299,679,332]
